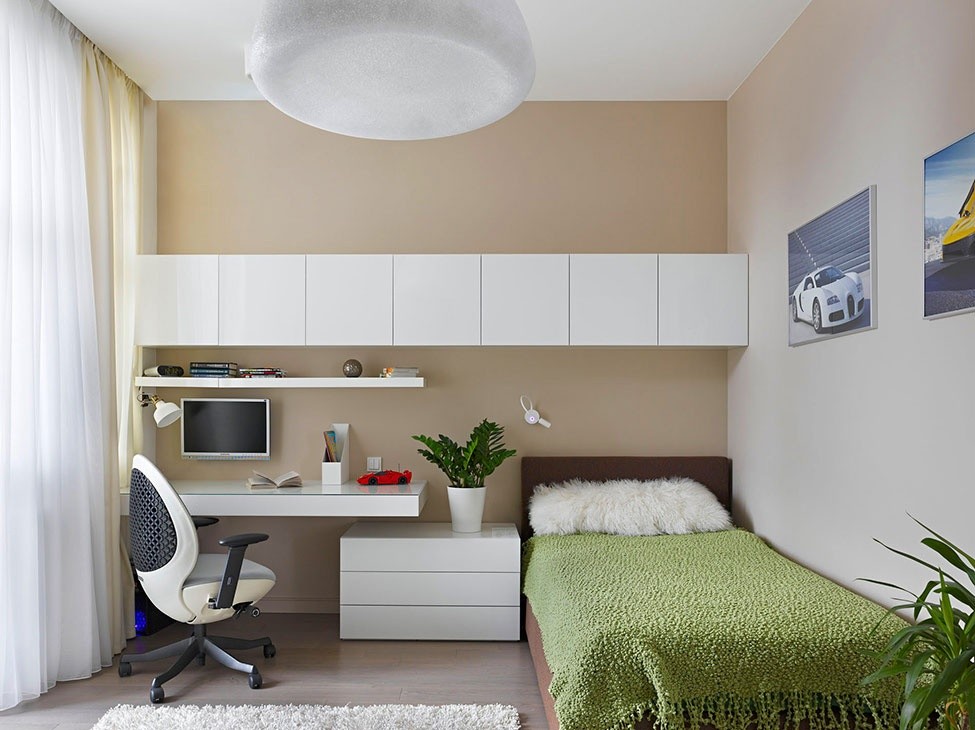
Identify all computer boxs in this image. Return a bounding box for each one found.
[129,557,178,636]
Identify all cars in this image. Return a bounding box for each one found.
[791,264,865,333]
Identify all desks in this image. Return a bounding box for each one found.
[120,478,429,516]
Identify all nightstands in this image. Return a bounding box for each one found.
[339,521,521,641]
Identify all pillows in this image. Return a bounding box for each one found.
[528,475,732,535]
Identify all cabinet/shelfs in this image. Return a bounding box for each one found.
[133,254,750,346]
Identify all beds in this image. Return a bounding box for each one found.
[520,455,968,730]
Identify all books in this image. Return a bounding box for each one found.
[380,367,419,378]
[246,470,303,489]
[189,362,288,378]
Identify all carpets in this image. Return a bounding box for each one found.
[88,703,521,730]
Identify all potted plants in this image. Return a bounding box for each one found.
[411,418,517,532]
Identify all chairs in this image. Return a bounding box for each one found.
[117,453,276,702]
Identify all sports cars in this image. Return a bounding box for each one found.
[357,463,412,486]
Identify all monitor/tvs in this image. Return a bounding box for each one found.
[181,398,271,460]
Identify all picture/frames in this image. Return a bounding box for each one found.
[786,185,878,346]
[921,128,975,321]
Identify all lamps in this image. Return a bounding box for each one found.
[136,393,182,428]
[242,1,534,140]
[520,395,551,429]
